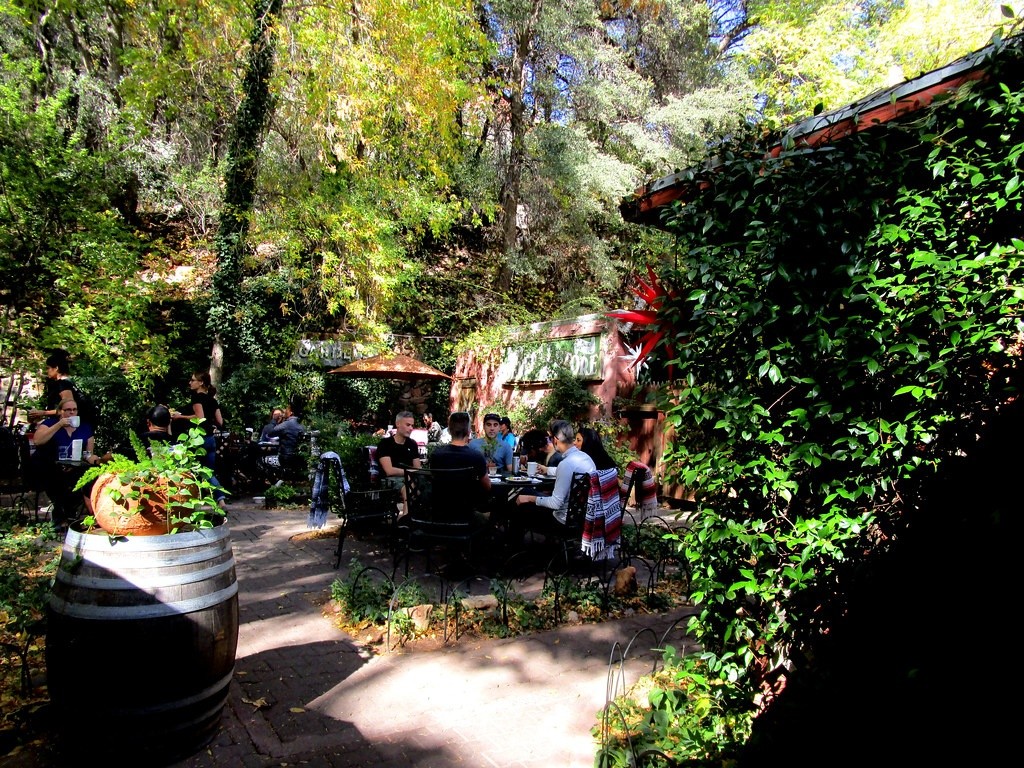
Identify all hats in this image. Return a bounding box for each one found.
[146,406,170,426]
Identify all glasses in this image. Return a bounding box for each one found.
[485,414,501,421]
[61,408,78,413]
[500,419,506,423]
[191,378,199,383]
[549,436,558,440]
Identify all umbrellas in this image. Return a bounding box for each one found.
[327,354,455,389]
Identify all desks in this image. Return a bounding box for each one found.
[487,473,556,570]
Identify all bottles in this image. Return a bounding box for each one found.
[512,453,520,476]
[83,451,91,459]
[519,441,528,471]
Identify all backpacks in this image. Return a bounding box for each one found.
[66,383,95,426]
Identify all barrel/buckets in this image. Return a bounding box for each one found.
[43,509,239,768]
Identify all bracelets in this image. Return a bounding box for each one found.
[94,460,98,464]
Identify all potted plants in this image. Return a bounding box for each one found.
[65,414,233,559]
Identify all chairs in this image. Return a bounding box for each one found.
[327,455,399,569]
[516,466,619,590]
[610,465,646,566]
[392,463,477,594]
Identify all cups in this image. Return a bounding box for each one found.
[69,416,80,427]
[58,445,68,460]
[412,458,421,468]
[526,462,539,473]
[489,466,497,475]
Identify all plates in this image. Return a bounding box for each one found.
[537,475,556,479]
[505,477,532,481]
[488,474,502,477]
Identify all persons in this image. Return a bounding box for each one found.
[255,402,304,483]
[26,353,228,534]
[375,410,617,580]
